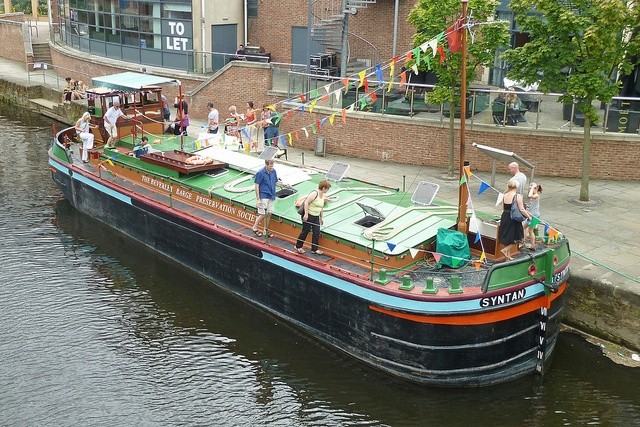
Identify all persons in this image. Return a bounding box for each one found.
[104,136,120,153]
[206,101,219,134]
[227,105,242,136]
[500,179,533,262]
[494,88,508,104]
[75,111,100,163]
[129,137,152,158]
[266,109,279,147]
[103,101,134,148]
[156,92,171,122]
[507,161,527,196]
[62,77,71,103]
[252,158,278,238]
[260,104,273,146]
[506,87,521,110]
[245,101,256,137]
[518,182,543,251]
[296,180,332,255]
[173,94,188,136]
[62,76,87,105]
[235,44,245,55]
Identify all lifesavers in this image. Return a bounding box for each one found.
[186,155,213,166]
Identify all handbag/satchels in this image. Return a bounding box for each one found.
[510,194,526,222]
[297,203,304,215]
[72,133,80,141]
[177,109,184,120]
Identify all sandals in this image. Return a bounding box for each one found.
[294,246,306,254]
[253,230,263,237]
[264,230,273,237]
[311,248,323,255]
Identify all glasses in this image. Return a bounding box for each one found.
[116,106,120,108]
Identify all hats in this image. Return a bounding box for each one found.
[78,81,83,84]
[207,102,213,107]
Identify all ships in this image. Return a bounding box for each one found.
[46,71,572,390]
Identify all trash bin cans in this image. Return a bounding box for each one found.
[603,95,640,134]
